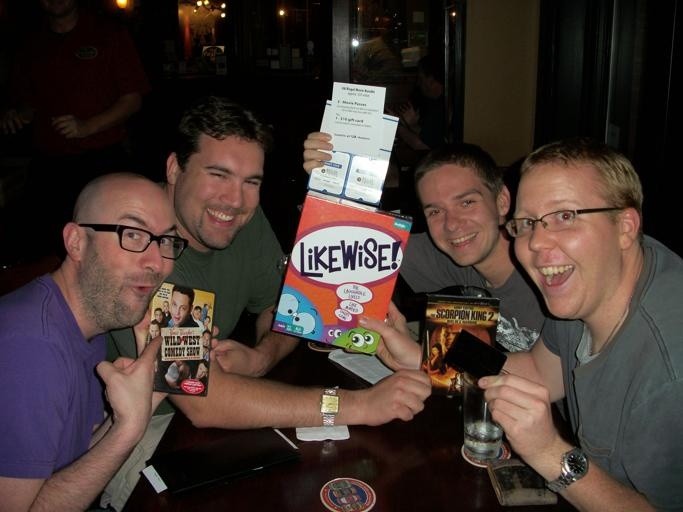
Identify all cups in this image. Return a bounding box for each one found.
[461,369,510,459]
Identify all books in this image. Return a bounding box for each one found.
[422,293,500,399]
[142,425,298,493]
[326,319,421,385]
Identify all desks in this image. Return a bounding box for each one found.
[114,348,584,511]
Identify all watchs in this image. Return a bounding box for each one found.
[544,446,590,496]
[319,386,339,428]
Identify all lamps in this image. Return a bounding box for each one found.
[193,0,227,19]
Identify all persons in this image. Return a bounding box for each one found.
[151,285,212,394]
[476,142,682,512]
[152,100,432,428]
[302,131,544,352]
[387,59,443,152]
[1,1,148,152]
[1,172,219,511]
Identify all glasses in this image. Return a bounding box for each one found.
[504,206,624,238]
[79,223,188,261]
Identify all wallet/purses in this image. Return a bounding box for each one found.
[487,459,558,506]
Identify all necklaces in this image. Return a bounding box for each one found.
[596,285,636,358]
